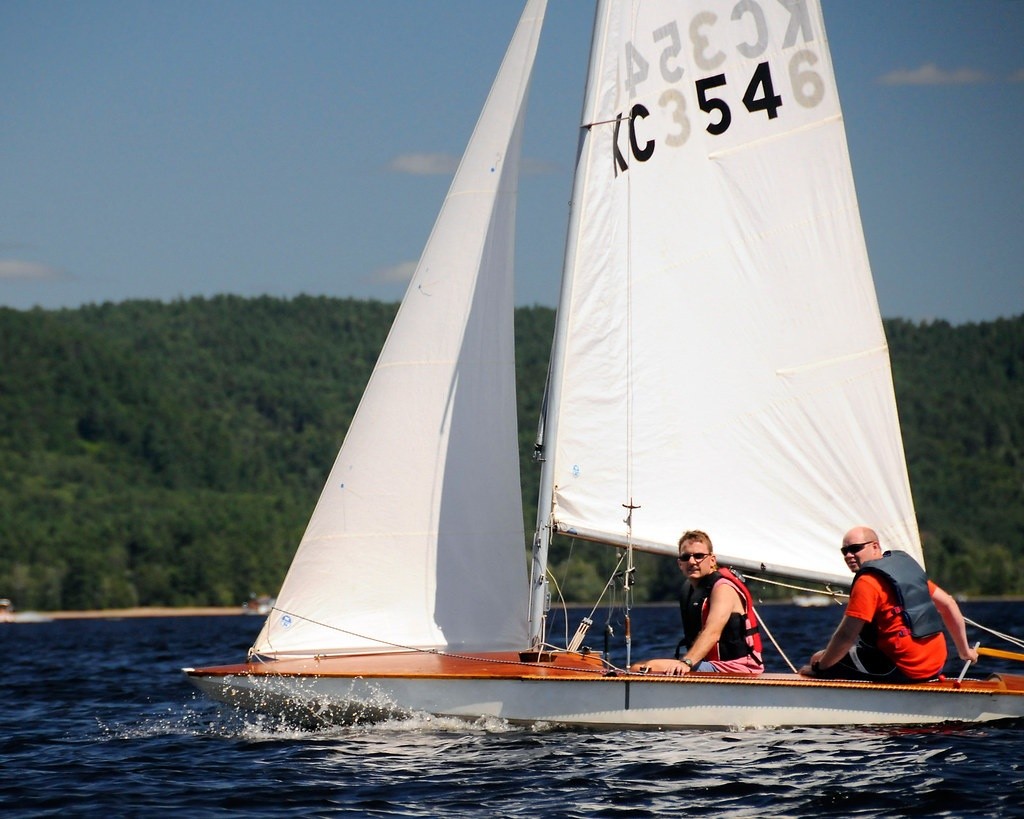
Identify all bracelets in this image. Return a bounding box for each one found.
[811,661,824,674]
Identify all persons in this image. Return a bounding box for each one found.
[798,526,978,684]
[631,531,765,676]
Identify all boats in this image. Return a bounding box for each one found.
[242,593,275,616]
[789,592,830,608]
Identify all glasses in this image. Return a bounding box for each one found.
[679,552,712,562]
[841,541,875,556]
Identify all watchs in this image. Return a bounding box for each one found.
[681,659,692,668]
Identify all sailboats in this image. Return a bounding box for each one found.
[175,0,1023,726]
